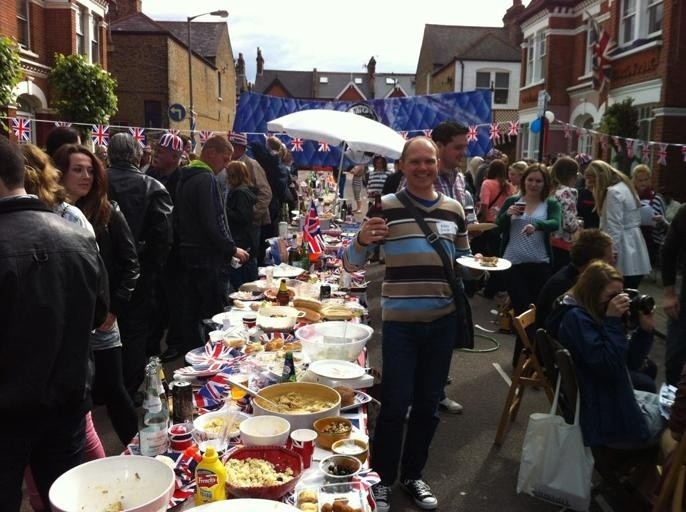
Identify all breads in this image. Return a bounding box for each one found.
[283,341,302,351]
[294,307,321,321]
[294,298,322,311]
[265,338,284,351]
[299,491,363,512]
[246,342,262,352]
[230,338,244,347]
[334,386,354,406]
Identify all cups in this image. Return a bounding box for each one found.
[318,455,362,491]
[168,423,195,453]
[514,201,527,220]
[290,428,318,469]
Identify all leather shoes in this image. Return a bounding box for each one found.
[163,347,181,362]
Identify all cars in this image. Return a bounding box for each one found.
[362,162,395,187]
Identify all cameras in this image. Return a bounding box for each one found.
[625,289,655,313]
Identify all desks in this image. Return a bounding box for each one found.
[74,180,376,512]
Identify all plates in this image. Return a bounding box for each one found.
[467,223,497,232]
[192,409,248,438]
[211,266,370,343]
[119,444,198,510]
[169,323,373,409]
[456,256,513,272]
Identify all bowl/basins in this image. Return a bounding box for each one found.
[223,445,303,496]
[47,453,176,512]
[239,414,291,446]
[312,417,352,448]
[250,381,341,429]
[330,439,369,464]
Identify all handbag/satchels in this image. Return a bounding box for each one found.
[516,372,595,511]
[499,308,515,334]
[456,277,474,349]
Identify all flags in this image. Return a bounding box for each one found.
[587,16,624,111]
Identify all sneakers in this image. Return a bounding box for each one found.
[439,396,464,414]
[399,479,438,510]
[372,484,392,512]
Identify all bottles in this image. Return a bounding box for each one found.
[291,171,356,237]
[288,235,311,271]
[194,444,225,505]
[139,353,171,456]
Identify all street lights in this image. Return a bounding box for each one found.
[187,10,229,153]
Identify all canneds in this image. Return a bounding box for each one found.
[320,284,331,300]
[173,382,193,424]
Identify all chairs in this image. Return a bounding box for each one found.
[495,303,686,512]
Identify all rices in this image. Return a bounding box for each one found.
[224,458,295,487]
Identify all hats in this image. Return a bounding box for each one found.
[151,132,183,152]
[228,133,248,146]
